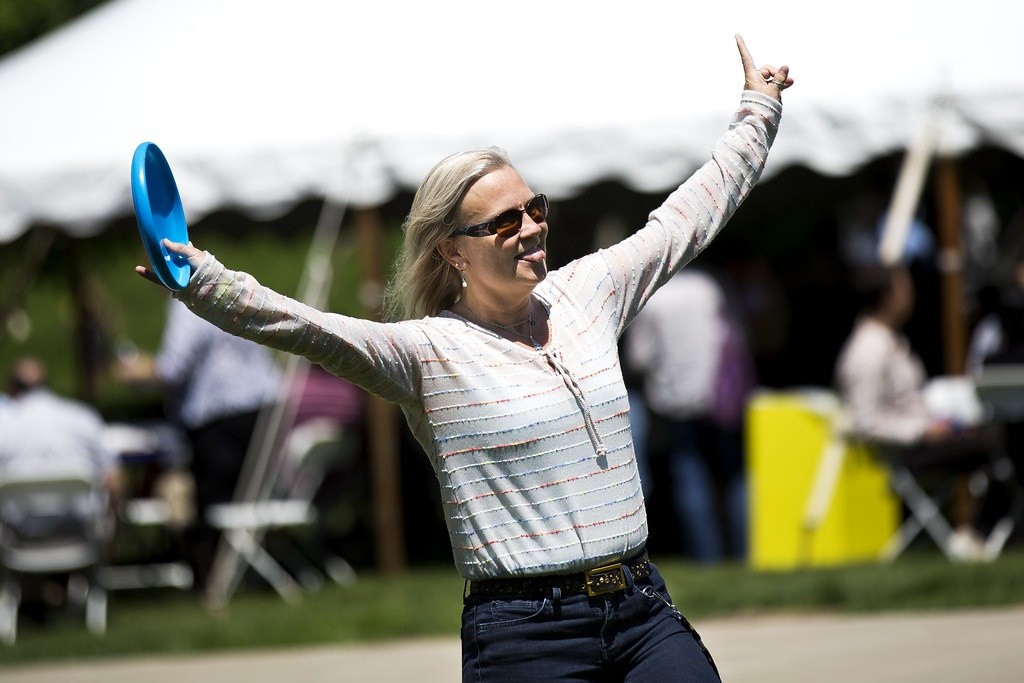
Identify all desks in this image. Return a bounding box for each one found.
[100,423,192,589]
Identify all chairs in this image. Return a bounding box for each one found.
[796,386,968,567]
[0,476,114,645]
[203,423,359,607]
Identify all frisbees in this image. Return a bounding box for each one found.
[128,141,192,292]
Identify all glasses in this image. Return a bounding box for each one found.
[448,194,550,238]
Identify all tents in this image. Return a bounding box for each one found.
[0,1,1024,577]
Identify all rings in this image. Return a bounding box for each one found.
[772,80,783,87]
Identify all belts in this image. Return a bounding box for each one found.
[470,548,650,597]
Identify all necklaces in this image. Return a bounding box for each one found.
[459,298,542,351]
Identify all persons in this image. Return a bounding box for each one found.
[835,167,1023,559]
[118,291,374,591]
[1,357,124,650]
[592,206,754,564]
[137,34,794,683]
